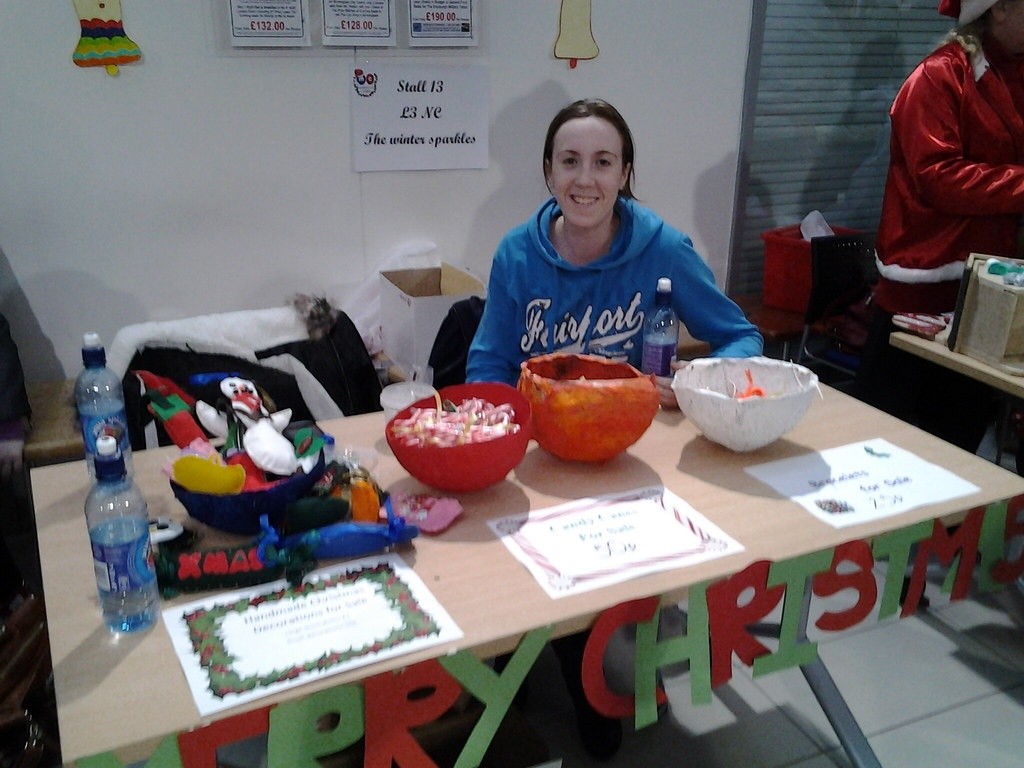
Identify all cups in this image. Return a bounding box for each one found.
[380,382,434,421]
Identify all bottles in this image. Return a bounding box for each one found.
[642,278,679,378]
[76,333,133,486]
[84,437,159,635]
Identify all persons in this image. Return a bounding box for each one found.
[863,0,1024,536]
[465,99,763,761]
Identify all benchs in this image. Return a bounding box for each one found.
[724,291,844,361]
[21,317,710,464]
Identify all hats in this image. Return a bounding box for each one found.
[938,0,999,26]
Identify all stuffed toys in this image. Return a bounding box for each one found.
[134,364,419,600]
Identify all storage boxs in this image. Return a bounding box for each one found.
[378,262,488,373]
[759,222,864,313]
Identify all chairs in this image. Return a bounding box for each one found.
[796,231,875,375]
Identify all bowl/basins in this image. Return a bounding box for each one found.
[671,358,818,452]
[170,446,325,535]
[385,383,531,493]
[518,352,660,464]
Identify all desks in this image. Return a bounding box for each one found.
[888,330,1024,475]
[29,383,1023,768]
